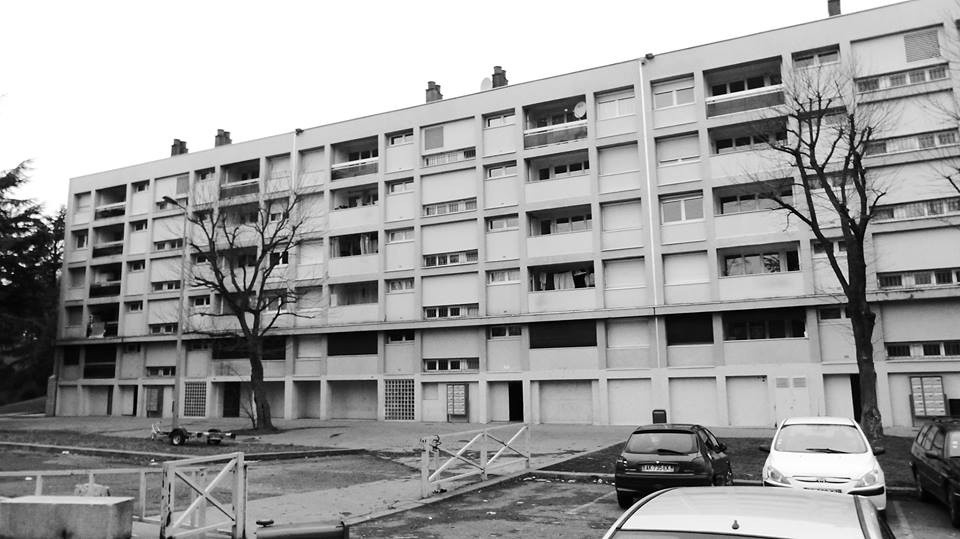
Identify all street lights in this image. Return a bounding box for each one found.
[163,195,190,429]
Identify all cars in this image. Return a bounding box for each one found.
[602,485,897,539]
[602,423,732,506]
[760,417,888,514]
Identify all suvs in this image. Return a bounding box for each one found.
[908,419,959,527]
